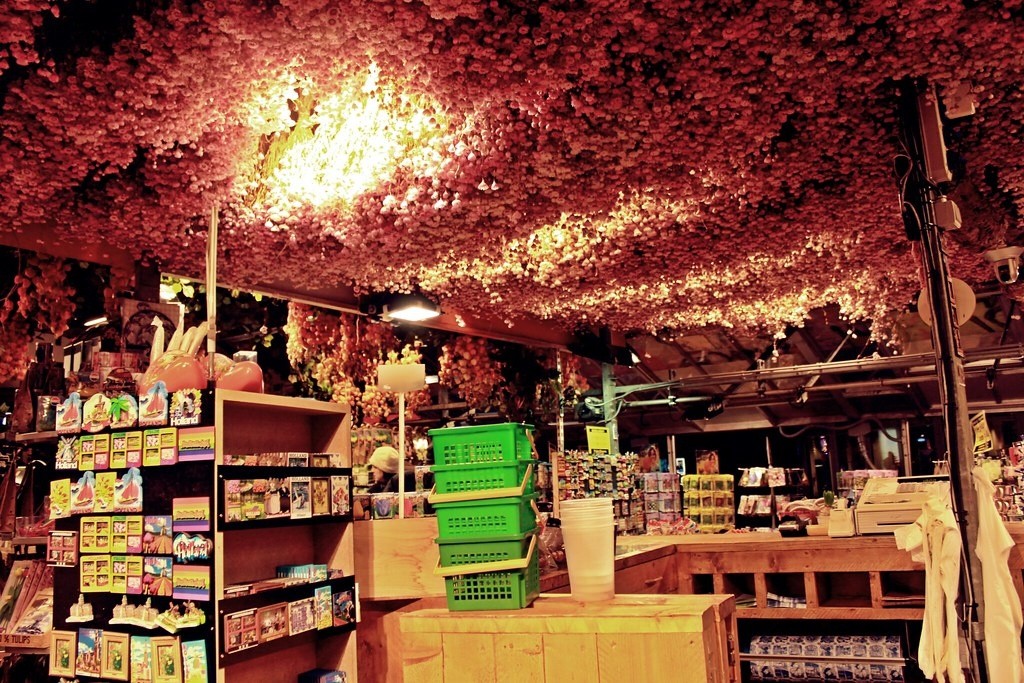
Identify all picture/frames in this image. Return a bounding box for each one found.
[49,629,76,678]
[151,636,183,683]
[101,632,130,682]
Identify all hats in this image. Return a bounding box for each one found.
[369,446,399,472]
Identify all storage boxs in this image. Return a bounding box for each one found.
[428,423,542,612]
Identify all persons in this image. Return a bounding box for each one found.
[365,445,399,493]
[988,419,1023,457]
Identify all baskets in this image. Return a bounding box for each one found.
[428,422,539,475]
[428,459,535,504]
[432,492,545,534]
[433,526,540,610]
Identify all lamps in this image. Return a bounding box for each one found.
[567,323,642,369]
[361,283,446,324]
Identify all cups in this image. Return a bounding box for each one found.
[559,497,616,600]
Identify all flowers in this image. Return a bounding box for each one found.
[0,1,1024,427]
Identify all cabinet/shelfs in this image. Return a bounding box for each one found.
[376,522,1024,683]
[0,388,358,683]
